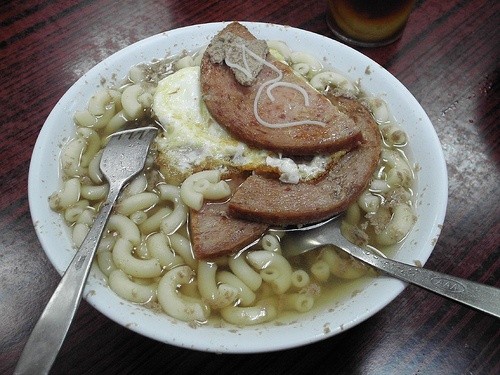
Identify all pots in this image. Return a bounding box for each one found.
[27,20,448,354]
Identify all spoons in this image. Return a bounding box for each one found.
[280,210,499,318]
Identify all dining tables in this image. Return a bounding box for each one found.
[1,0,499,375]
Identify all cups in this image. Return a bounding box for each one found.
[326,0,416,49]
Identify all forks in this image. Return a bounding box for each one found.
[15,118,160,374]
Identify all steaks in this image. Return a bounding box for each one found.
[187,21,382,259]
